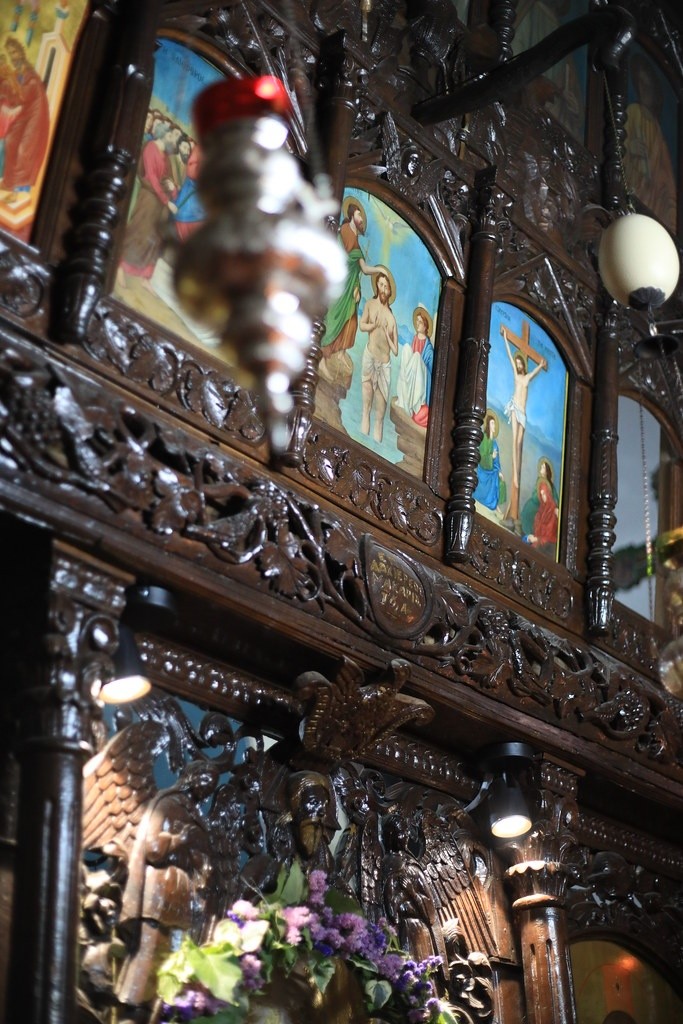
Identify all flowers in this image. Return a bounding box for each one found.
[163,870,448,1018]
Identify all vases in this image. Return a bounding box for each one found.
[283,959,364,1024]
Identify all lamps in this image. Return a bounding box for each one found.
[596,70,679,336]
[466,743,534,838]
[97,586,169,704]
